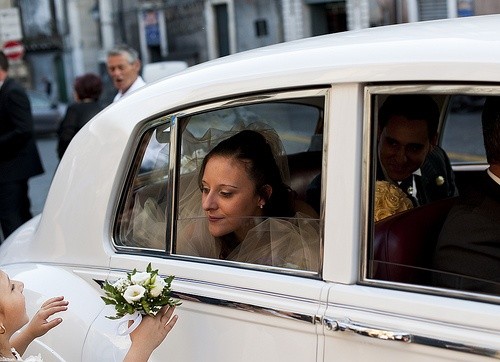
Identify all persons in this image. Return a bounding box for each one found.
[177,129,321,273]
[57,73,111,161]
[1,270,177,361]
[105,44,147,103]
[0,48,47,241]
[432,94,500,297]
[303,93,460,215]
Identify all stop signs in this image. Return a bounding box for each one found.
[2,40,25,61]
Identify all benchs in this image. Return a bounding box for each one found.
[149,146,341,277]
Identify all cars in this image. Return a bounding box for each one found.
[0,13,499,360]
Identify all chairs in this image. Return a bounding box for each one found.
[362,195,466,291]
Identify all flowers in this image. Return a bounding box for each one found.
[99,265,182,325]
[374,181,414,225]
